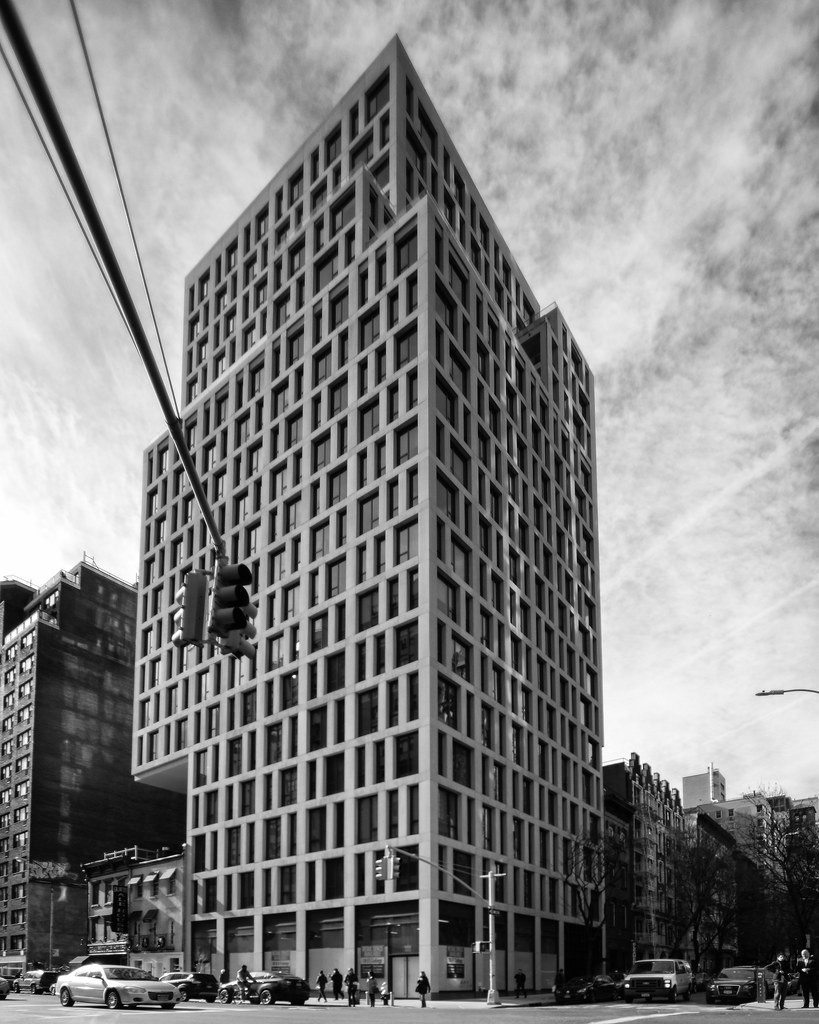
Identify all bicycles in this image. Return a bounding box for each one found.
[231,982,269,1006]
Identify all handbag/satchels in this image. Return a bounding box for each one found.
[772,971,784,982]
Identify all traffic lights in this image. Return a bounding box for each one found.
[390,856,401,880]
[171,572,207,650]
[475,941,486,953]
[208,560,253,640]
[375,857,389,880]
[219,601,258,660]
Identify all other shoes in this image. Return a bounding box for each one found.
[780,1006,788,1009]
[774,1007,781,1011]
[524,995,527,998]
[515,996,519,999]
[813,1005,819,1008]
[802,1004,809,1008]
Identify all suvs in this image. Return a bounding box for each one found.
[13,970,60,995]
[622,959,693,1004]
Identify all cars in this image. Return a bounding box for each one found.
[691,964,800,1004]
[54,963,182,1010]
[553,974,616,1006]
[606,970,625,1000]
[158,971,220,1003]
[0,976,11,1000]
[218,971,310,1006]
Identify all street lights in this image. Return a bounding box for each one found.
[774,829,800,953]
[15,857,54,970]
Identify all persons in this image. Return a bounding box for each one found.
[236,965,257,999]
[554,969,564,993]
[767,953,789,1010]
[514,969,528,999]
[146,971,153,979]
[316,970,328,1003]
[366,971,389,1007]
[15,972,20,978]
[220,969,227,986]
[329,968,344,1000]
[797,949,819,1008]
[344,968,359,1007]
[415,971,430,1008]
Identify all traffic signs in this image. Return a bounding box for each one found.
[487,908,500,916]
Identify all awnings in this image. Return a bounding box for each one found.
[128,909,158,922]
[128,868,175,884]
[69,956,90,966]
